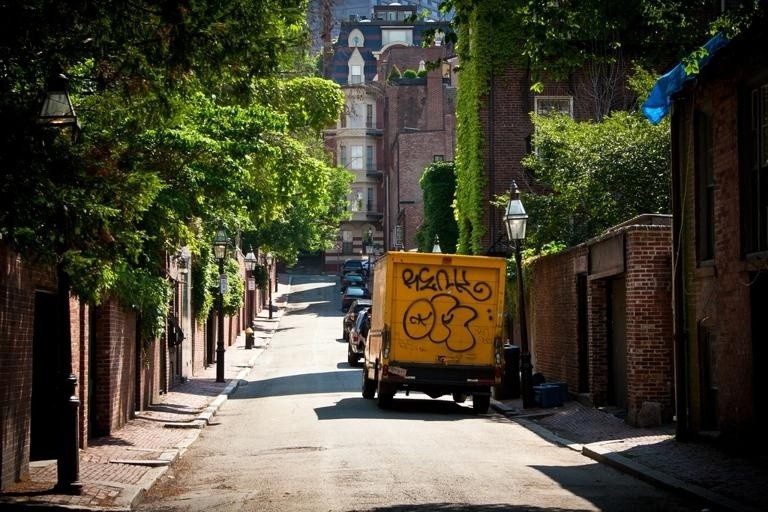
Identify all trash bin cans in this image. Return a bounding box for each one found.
[532,384,569,408]
[492,346,521,401]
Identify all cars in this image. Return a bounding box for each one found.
[338,256,373,370]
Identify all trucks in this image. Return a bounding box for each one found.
[358,250,508,414]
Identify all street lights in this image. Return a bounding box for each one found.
[243,242,259,351]
[429,234,443,253]
[500,178,539,413]
[367,226,373,275]
[35,45,91,500]
[211,216,232,386]
[266,251,273,321]
